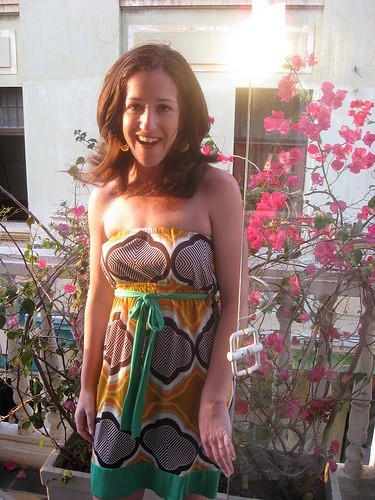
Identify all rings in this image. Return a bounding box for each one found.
[217,445,225,450]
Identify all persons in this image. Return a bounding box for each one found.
[73,44,250,500]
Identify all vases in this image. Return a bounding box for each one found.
[39,428,341,500]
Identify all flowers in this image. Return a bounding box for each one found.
[8,52,374,482]
